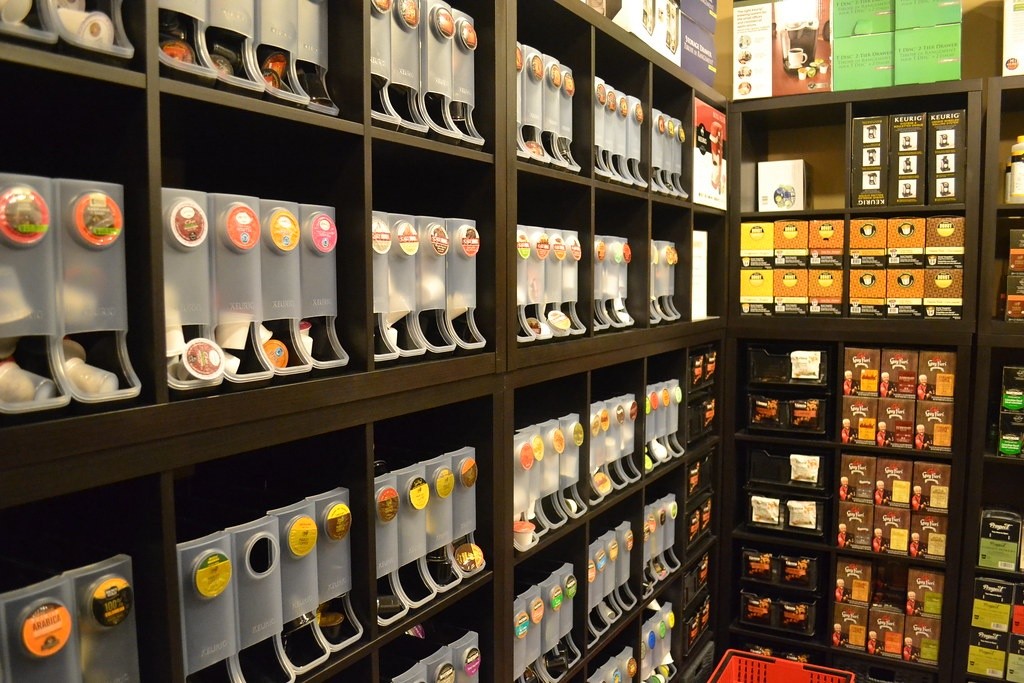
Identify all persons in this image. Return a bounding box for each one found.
[831,576,925,663]
[845,368,936,450]
[838,475,931,559]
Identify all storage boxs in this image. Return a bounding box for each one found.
[605,0,1024,683]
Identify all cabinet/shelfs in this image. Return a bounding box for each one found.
[949,72,1024,683]
[508,0,727,683]
[727,77,983,683]
[0,0,508,683]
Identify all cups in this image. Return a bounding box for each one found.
[788,47,807,68]
